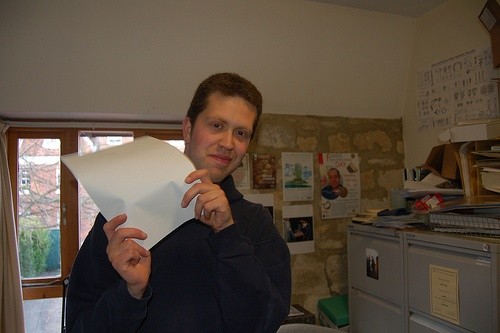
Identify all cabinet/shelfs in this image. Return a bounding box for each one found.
[347,222,500,333]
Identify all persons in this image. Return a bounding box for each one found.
[65,72,292,333]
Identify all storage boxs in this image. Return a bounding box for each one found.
[284,303,316,326]
[319,295,350,333]
[424,120,500,207]
